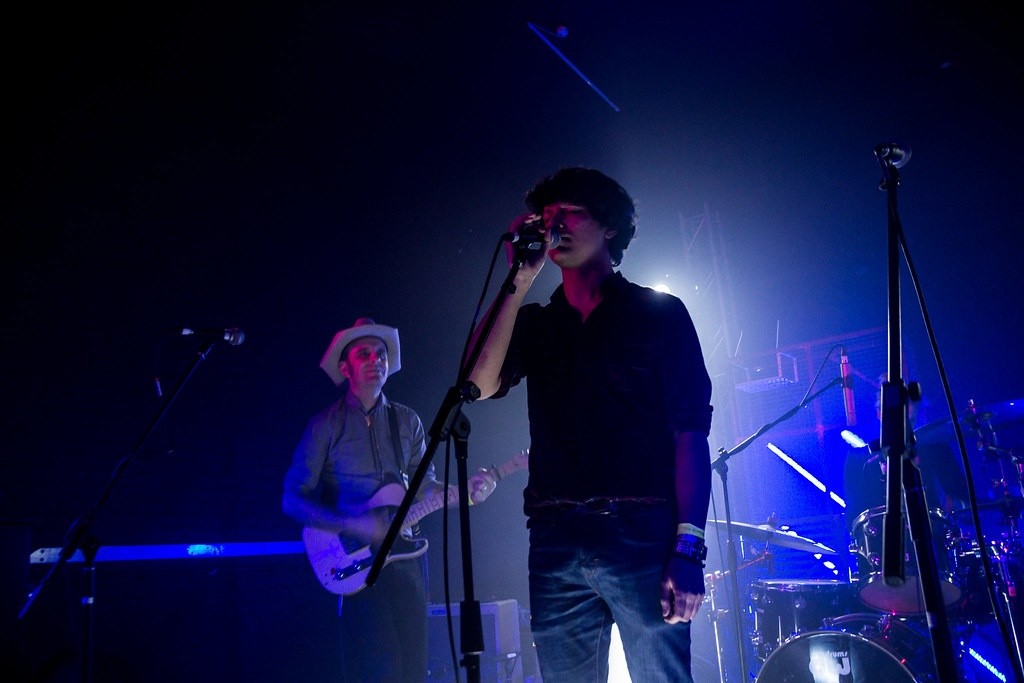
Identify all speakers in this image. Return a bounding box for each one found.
[425,599,525,683]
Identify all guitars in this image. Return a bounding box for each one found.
[302,448,529,595]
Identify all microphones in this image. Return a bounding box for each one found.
[503,227,561,250]
[182,327,245,346]
[840,347,858,427]
[873,142,913,168]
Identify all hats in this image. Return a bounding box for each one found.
[319,318,404,388]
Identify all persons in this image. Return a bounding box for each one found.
[466,169,714,683]
[844,372,969,573]
[281,318,496,683]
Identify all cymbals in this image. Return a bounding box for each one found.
[706,519,840,555]
[956,496,1024,512]
[914,399,1024,450]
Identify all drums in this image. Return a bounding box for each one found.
[747,579,848,662]
[851,504,970,616]
[753,614,966,683]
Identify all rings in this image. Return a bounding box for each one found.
[482,486,487,491]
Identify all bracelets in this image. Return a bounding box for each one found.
[468,494,474,506]
[674,522,707,566]
